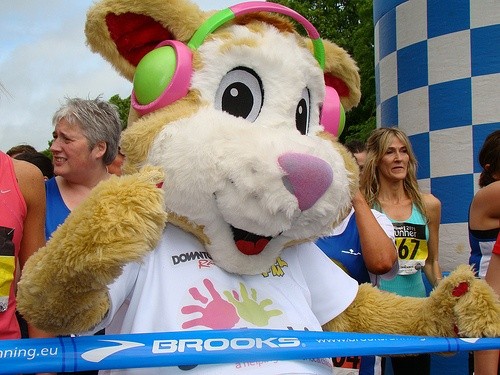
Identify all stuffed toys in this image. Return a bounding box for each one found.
[22,0,500,375]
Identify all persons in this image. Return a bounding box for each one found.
[470,134,499,374]
[358,125,448,301]
[42,97,124,240]
[1,148,52,341]
